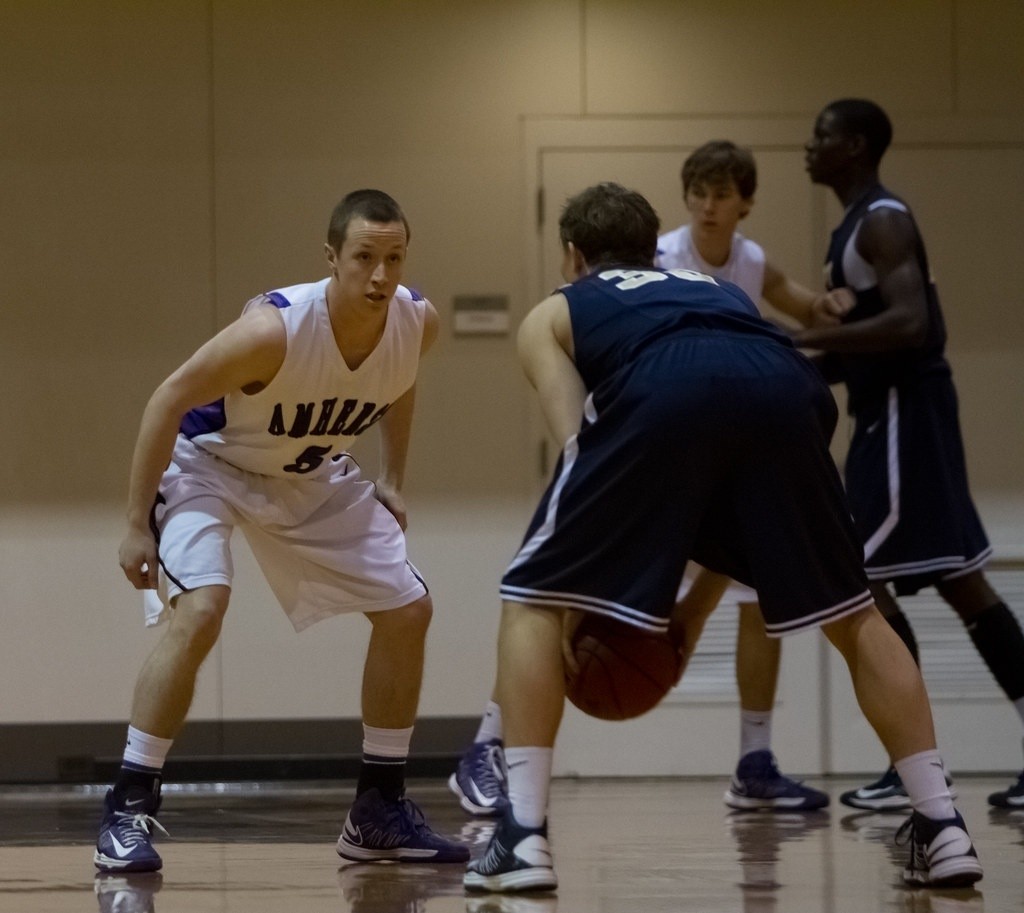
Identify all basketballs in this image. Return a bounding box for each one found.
[559,604,686,726]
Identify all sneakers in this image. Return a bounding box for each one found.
[723,749,829,810]
[894,806,983,885]
[840,761,959,811]
[447,736,510,816]
[988,769,1024,807]
[94,789,170,872]
[335,786,470,863]
[461,800,558,893]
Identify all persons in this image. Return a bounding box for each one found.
[781,96,1023,813]
[463,181,984,890]
[92,190,472,877]
[450,137,856,813]
